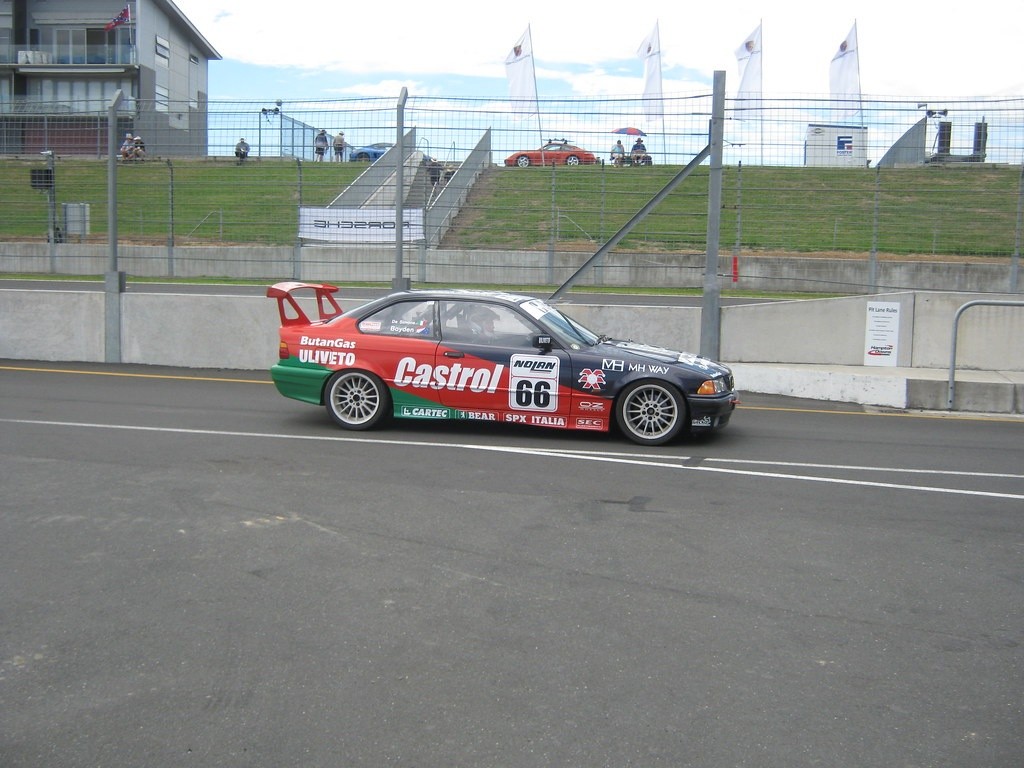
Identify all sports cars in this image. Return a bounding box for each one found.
[261,283,741,446]
[503,139,597,168]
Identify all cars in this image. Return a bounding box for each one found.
[349,142,396,161]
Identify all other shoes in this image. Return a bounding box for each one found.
[614,165,617,168]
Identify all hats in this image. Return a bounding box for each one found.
[339,131,344,135]
[447,165,455,172]
[134,136,141,141]
[635,138,643,142]
[431,157,438,162]
[126,134,133,139]
[320,130,327,133]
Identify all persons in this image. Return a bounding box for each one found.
[235,138,250,166]
[611,140,624,167]
[427,156,443,186]
[120,133,146,161]
[333,131,345,162]
[631,138,646,163]
[444,165,455,185]
[314,130,329,162]
[465,306,500,339]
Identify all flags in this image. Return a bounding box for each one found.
[828,23,860,122]
[636,22,664,121]
[505,26,538,120]
[733,23,761,119]
[104,4,129,32]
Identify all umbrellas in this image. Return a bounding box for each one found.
[612,127,647,156]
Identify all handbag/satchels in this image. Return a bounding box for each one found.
[317,142,326,148]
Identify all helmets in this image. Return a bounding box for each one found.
[460,305,500,343]
